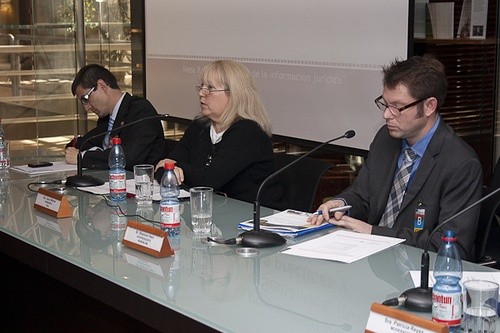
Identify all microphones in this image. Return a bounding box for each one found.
[66,113,170,187]
[238,130,355,248]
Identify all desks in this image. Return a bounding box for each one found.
[0,158,500,333]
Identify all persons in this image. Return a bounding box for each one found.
[153,60,288,211]
[65,64,164,173]
[307,56,483,261]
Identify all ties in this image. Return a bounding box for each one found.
[378,148,418,228]
[103,119,114,150]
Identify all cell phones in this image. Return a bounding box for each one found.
[28,162,53,168]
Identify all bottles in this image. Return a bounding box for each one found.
[431,229,463,333]
[0,121,6,170]
[109,200,128,258]
[161,237,181,304]
[108,138,126,201]
[159,161,181,237]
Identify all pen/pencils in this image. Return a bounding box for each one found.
[313,205,351,215]
[72,138,77,147]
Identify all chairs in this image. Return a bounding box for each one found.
[269,154,335,214]
[463,155,500,271]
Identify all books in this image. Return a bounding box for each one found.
[236,208,335,239]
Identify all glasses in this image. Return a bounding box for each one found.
[373,94,433,116]
[195,85,230,95]
[79,85,96,105]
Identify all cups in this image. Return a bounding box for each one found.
[191,241,211,278]
[136,208,153,229]
[0,141,10,170]
[462,280,500,333]
[190,187,213,237]
[133,164,154,205]
[0,170,11,205]
